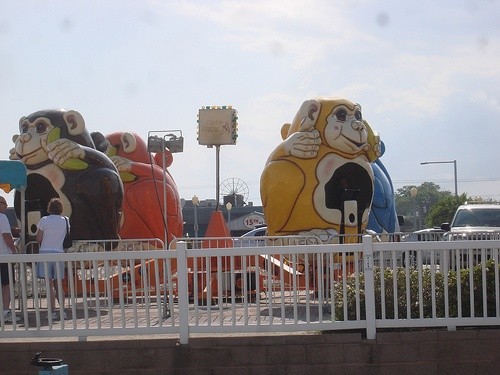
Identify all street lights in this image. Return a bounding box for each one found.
[410,187,419,229]
[226,202,233,235]
[192,194,199,247]
[419,160,459,202]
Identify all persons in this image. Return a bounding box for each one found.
[36,198,73,320]
[0,196,21,322]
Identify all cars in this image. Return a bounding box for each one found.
[401,226,447,269]
[231,226,266,247]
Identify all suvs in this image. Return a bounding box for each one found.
[440,198,500,271]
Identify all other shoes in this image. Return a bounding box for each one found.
[59,312,67,321]
[0,312,21,322]
[45,312,56,320]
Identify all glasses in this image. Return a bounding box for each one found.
[0,201,8,206]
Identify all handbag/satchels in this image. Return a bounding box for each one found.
[64,215,72,249]
